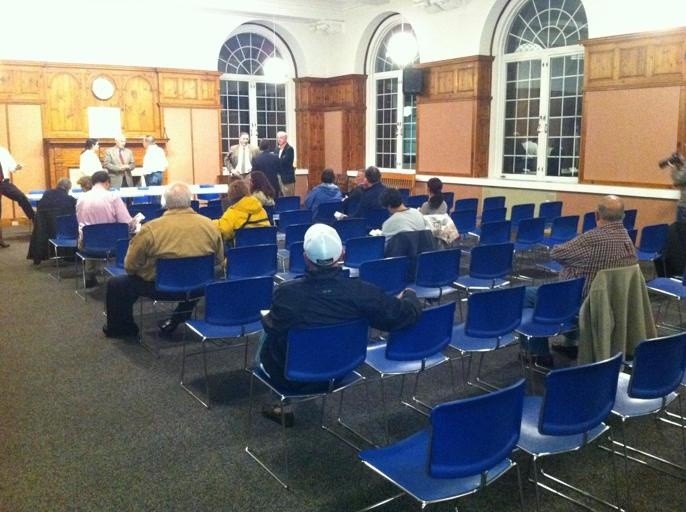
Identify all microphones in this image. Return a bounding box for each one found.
[225,151,232,160]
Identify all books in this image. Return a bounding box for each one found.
[370,229,382,236]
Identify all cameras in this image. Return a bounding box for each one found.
[659,153,684,169]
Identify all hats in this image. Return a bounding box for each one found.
[303,223,343,266]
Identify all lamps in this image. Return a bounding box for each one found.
[384,1,423,69]
[261,3,292,85]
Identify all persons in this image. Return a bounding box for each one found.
[420,176,448,215]
[223,131,255,183]
[103,181,227,338]
[79,138,108,177]
[25,177,76,265]
[518,193,639,366]
[670,141,686,221]
[303,167,342,220]
[273,131,296,196]
[74,170,137,288]
[141,135,168,185]
[377,186,427,254]
[245,170,277,208]
[250,222,421,426]
[336,166,392,220]
[102,132,136,188]
[251,139,283,196]
[343,167,369,209]
[77,176,92,191]
[504,130,567,178]
[0,144,36,248]
[211,179,271,247]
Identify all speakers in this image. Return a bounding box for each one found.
[403,69,426,96]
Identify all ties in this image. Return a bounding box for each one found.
[119,148,125,175]
[241,146,245,174]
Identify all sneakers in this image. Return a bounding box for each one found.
[0,239,10,247]
[86,278,97,288]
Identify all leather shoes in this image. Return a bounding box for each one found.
[261,402,294,427]
[103,316,178,338]
[519,339,578,372]
